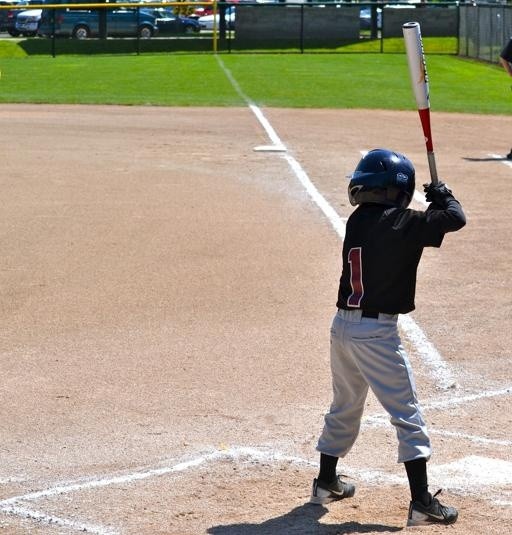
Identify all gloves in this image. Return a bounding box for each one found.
[422,180,454,202]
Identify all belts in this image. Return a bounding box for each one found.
[361,311,378,319]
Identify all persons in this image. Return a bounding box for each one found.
[307,147,467,528]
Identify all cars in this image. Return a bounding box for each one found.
[190,5,235,30]
[38,5,160,40]
[140,8,202,36]
[16,8,48,34]
[1,1,26,37]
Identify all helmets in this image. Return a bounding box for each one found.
[348,149,415,208]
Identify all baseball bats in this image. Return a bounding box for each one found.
[403,21,439,184]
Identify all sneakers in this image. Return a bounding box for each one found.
[311,476,355,505]
[407,490,458,525]
